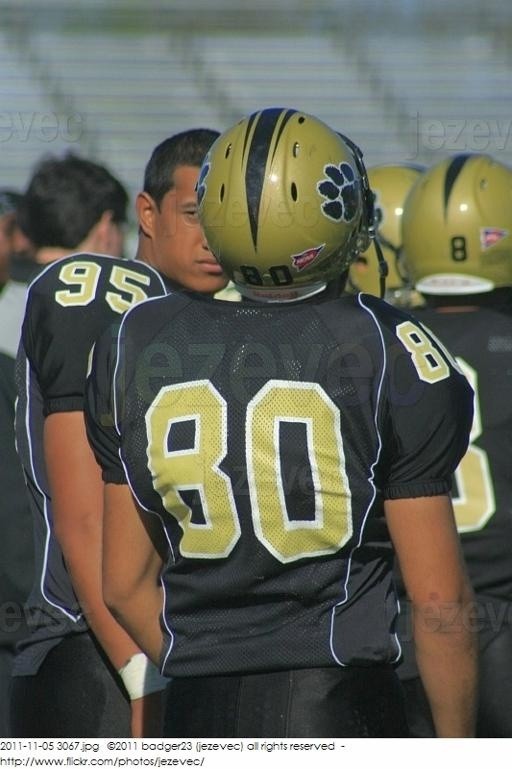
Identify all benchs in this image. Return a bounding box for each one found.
[0,28,510,190]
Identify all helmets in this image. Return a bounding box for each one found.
[198,108,510,305]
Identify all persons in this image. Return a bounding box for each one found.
[1,107,512,738]
